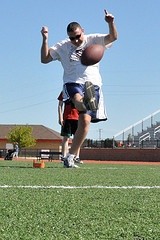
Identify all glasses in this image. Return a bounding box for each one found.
[69,30,82,40]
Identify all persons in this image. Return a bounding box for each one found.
[13,141,19,159]
[83,139,138,148]
[5,149,14,160]
[41,9,118,167]
[57,91,83,164]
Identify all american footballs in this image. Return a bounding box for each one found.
[79,44,105,66]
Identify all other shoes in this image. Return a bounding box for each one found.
[61,157,65,161]
[80,81,98,112]
[64,156,78,168]
[73,157,83,164]
